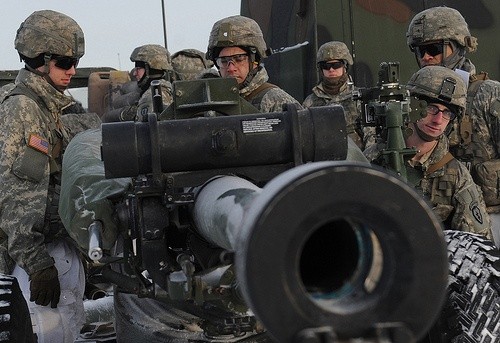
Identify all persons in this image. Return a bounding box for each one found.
[407,5,500,207]
[302,40,376,150]
[206,16,304,113]
[0,10,103,343]
[131,45,173,122]
[363,66,490,241]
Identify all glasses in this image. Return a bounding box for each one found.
[425,104,457,121]
[135,61,149,69]
[322,61,345,70]
[45,54,80,70]
[216,53,248,69]
[415,42,444,59]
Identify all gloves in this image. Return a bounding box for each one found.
[29,267,61,308]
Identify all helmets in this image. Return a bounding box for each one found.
[14,9,85,58]
[406,7,478,53]
[316,41,354,73]
[206,15,274,61]
[131,44,173,72]
[171,50,214,81]
[406,66,467,119]
